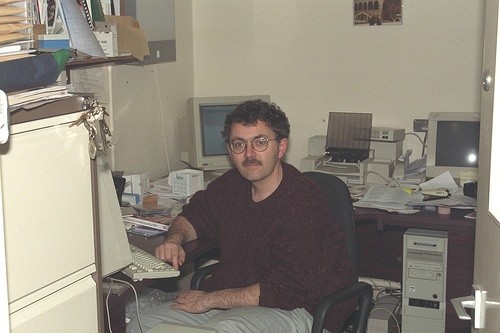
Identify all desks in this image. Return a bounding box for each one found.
[102,188,477,333]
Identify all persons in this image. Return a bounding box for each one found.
[124,99,359,333]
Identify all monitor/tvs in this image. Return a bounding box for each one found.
[178,95,270,176]
[426,112,481,186]
[95,153,134,280]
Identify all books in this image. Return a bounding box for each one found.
[125,208,179,231]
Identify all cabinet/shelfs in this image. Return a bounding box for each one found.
[0,109,100,332]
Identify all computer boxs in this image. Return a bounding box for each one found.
[401,227,448,333]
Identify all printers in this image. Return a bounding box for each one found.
[301,112,412,188]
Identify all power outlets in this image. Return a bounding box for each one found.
[413,119,428,132]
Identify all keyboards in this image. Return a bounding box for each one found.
[121,244,181,281]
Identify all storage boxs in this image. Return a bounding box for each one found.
[37,33,69,49]
[171,168,205,195]
[122,169,150,205]
[93,21,119,57]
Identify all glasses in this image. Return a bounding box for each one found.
[229,137,282,154]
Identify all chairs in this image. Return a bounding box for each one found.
[189,172,373,333]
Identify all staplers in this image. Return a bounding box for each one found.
[421,189,451,201]
